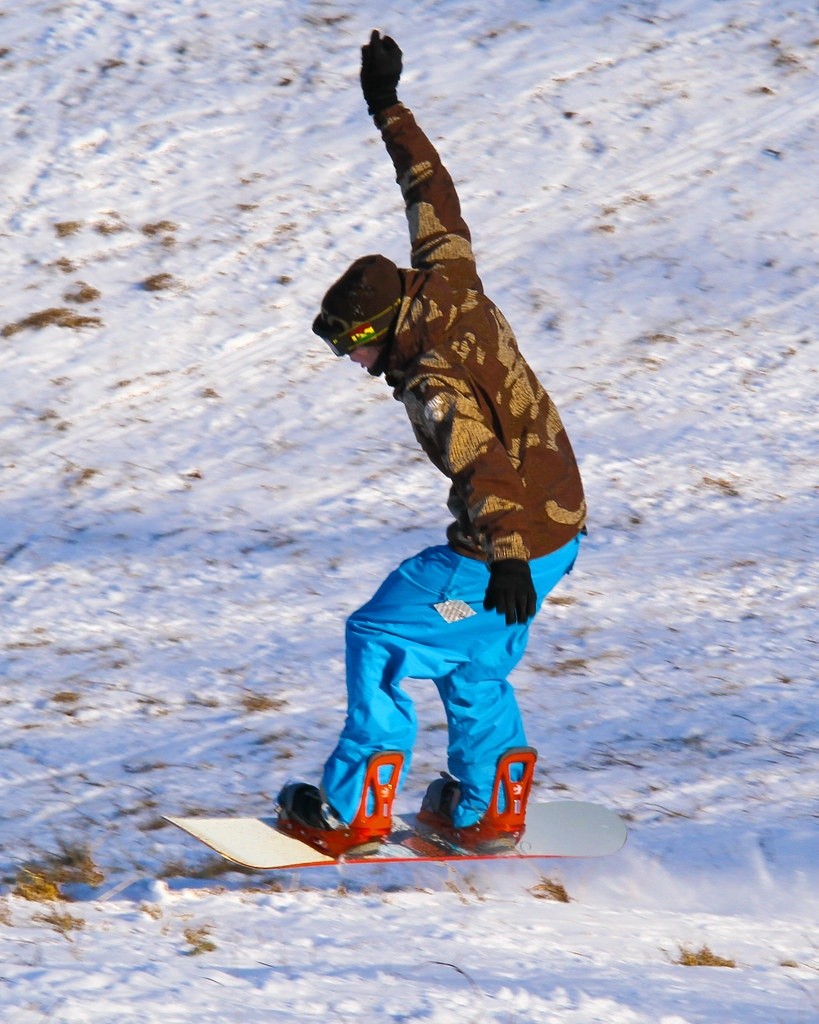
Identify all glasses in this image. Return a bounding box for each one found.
[312,313,363,355]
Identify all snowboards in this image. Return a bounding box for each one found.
[159,799,629,871]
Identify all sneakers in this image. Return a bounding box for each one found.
[281,782,377,855]
[428,776,515,855]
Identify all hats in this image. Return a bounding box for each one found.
[322,254,401,345]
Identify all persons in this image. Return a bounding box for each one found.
[276,27,592,863]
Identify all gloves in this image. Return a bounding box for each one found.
[484,559,537,627]
[361,29,402,116]
